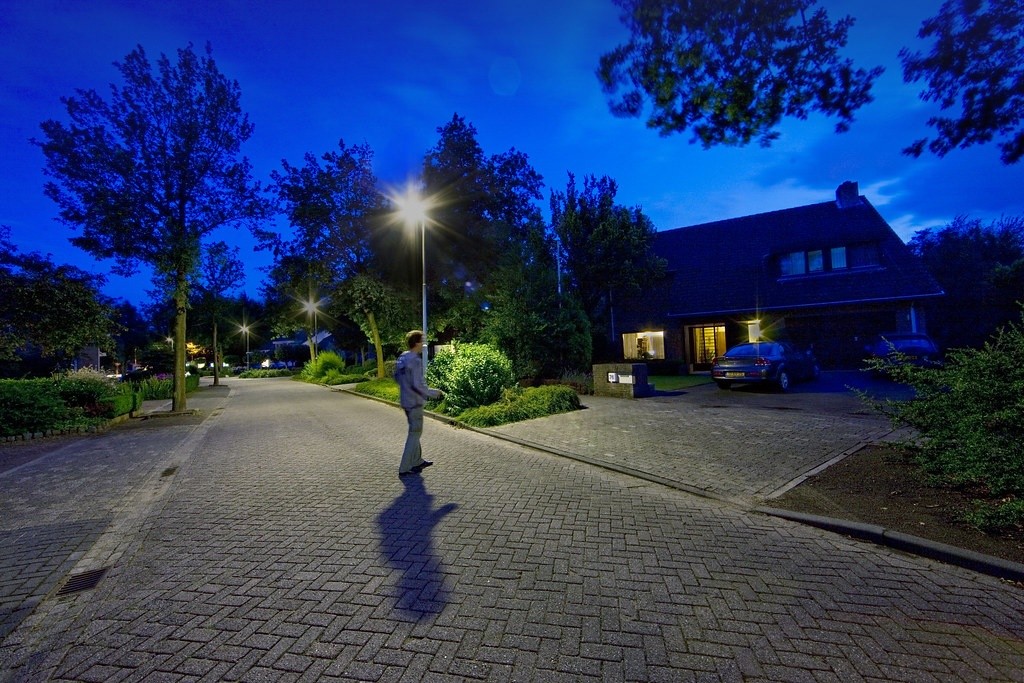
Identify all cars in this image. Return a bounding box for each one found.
[864,331,941,381]
[710,339,824,394]
[262,358,287,369]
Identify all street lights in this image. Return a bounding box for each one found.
[167,338,174,351]
[402,193,429,382]
[242,327,250,372]
[306,301,318,369]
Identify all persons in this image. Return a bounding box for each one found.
[395,330,444,474]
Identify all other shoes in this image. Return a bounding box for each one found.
[419,461,434,467]
[399,471,415,477]
